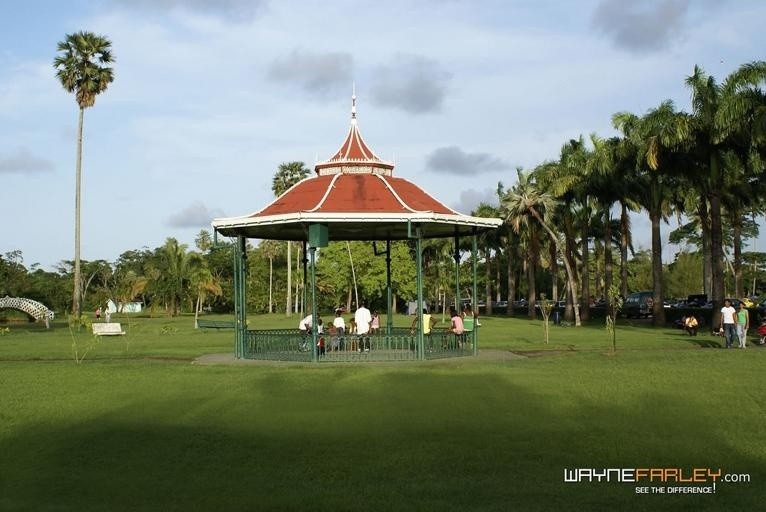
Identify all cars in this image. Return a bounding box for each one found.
[744,298,759,307]
[459,291,746,317]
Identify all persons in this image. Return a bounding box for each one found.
[297,301,383,354]
[720,299,737,348]
[447,309,465,335]
[684,314,698,335]
[95,307,101,320]
[410,307,438,336]
[735,302,751,347]
[105,306,111,322]
[462,304,475,317]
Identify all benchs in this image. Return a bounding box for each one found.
[196,318,248,333]
[91,322,131,352]
